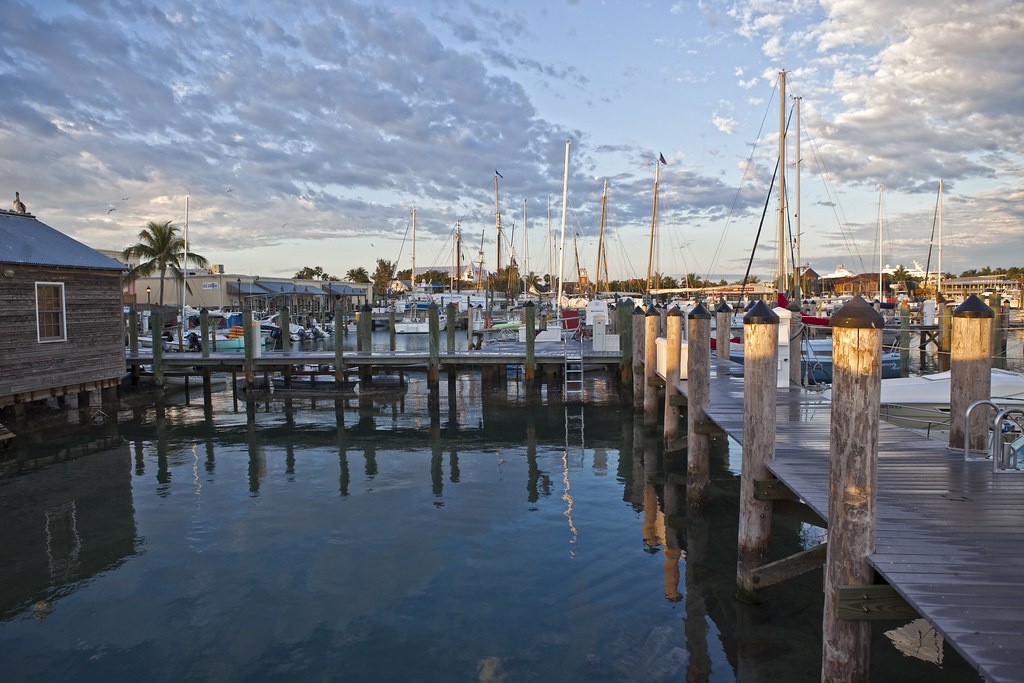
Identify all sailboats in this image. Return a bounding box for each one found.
[126,65,1024,426]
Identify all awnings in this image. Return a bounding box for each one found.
[227,280,368,297]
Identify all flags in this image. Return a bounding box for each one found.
[495,170,504,179]
[660,153,667,165]
